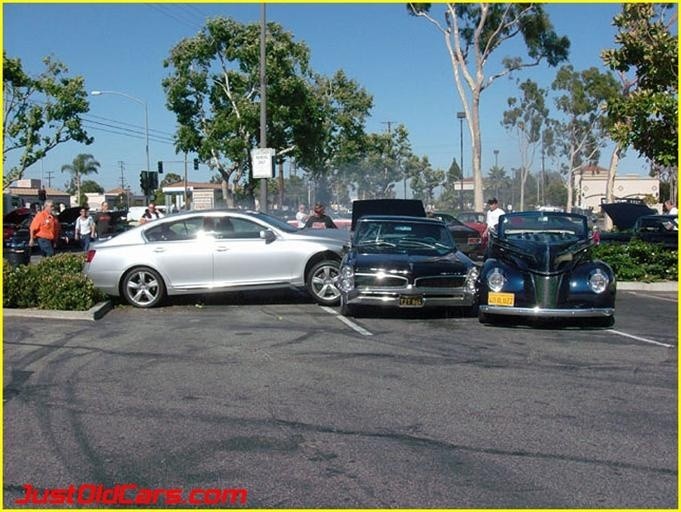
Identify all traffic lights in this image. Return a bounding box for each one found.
[158,161,163,174]
[194,158,199,170]
[140,171,147,191]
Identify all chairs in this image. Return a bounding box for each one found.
[148,232,164,240]
[163,229,178,238]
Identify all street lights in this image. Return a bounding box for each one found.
[456,112,467,213]
[90,90,153,205]
[493,149,500,201]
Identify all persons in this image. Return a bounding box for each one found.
[482,197,509,262]
[74,208,96,253]
[295,203,309,230]
[91,202,114,242]
[28,199,59,258]
[300,204,338,230]
[664,200,678,231]
[140,203,166,225]
